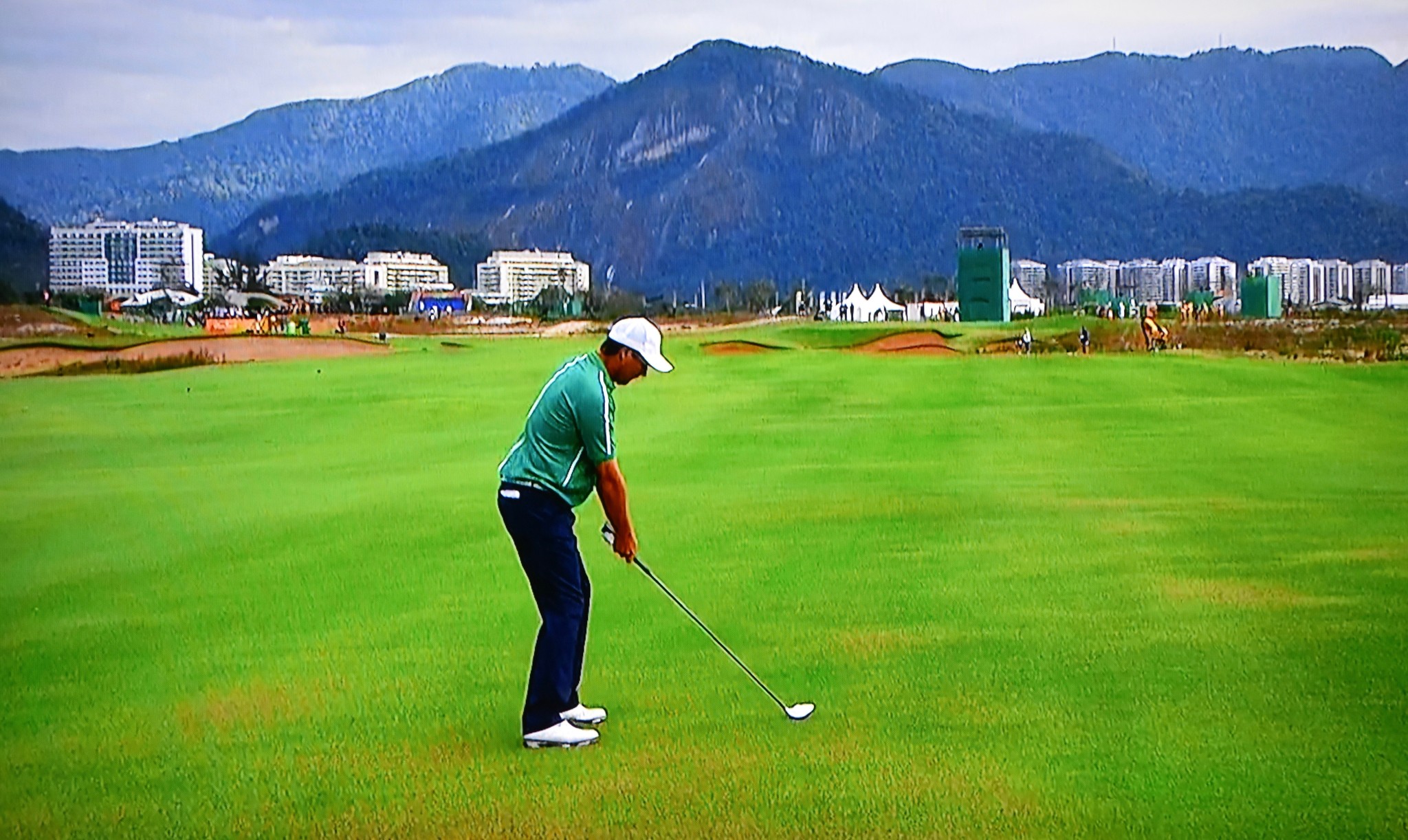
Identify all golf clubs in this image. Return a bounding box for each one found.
[599,523,814,720]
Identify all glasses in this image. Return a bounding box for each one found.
[625,347,648,378]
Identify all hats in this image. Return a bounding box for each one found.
[609,316,675,373]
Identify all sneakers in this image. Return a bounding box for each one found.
[523,719,600,749]
[559,703,608,723]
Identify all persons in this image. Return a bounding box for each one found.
[1095,293,1300,352]
[799,297,961,323]
[1021,326,1034,356]
[1078,325,1089,354]
[498,315,675,748]
[1015,338,1022,355]
[45,288,52,307]
[156,299,436,337]
